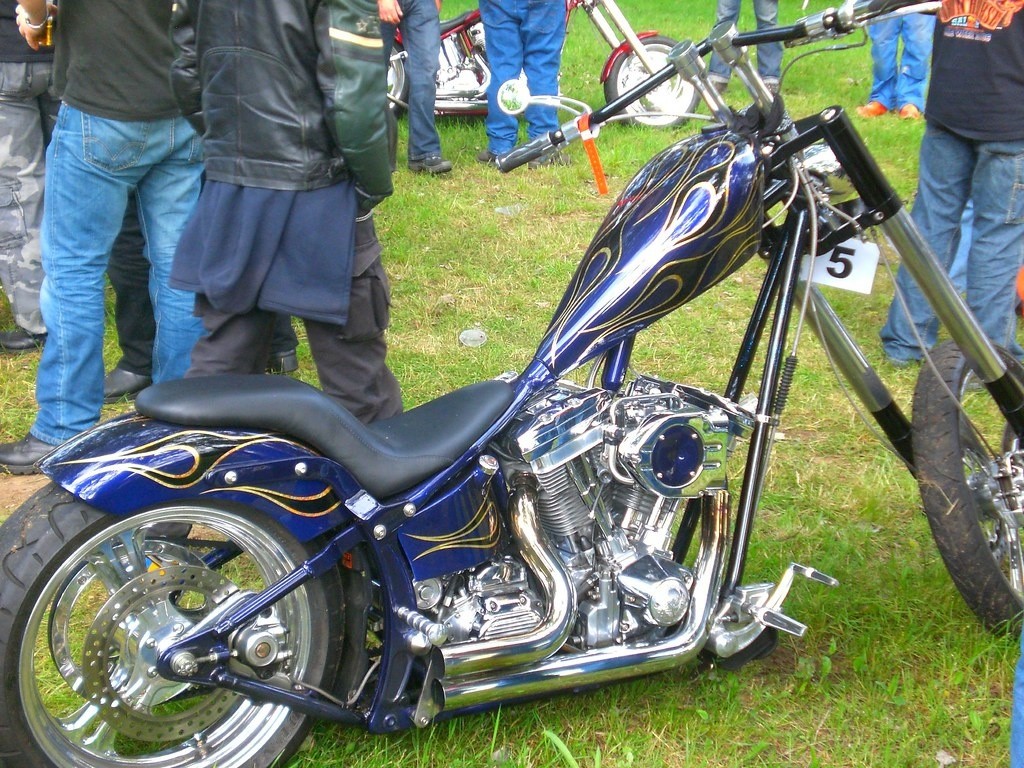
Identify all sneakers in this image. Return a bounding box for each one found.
[899,104,920,119]
[856,100,888,119]
[408,154,453,173]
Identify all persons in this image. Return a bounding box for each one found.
[0,0,568,475]
[709,0,784,97]
[855,0,1024,393]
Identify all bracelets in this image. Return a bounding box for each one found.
[25,6,49,29]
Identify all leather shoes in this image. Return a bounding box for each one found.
[0,433,57,474]
[265,349,298,374]
[0,330,48,354]
[104,367,152,403]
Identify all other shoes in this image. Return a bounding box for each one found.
[528,159,540,169]
[477,150,498,167]
[763,76,779,96]
[711,79,727,94]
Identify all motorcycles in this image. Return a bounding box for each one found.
[382,0,704,131]
[0,0,1024,768]
